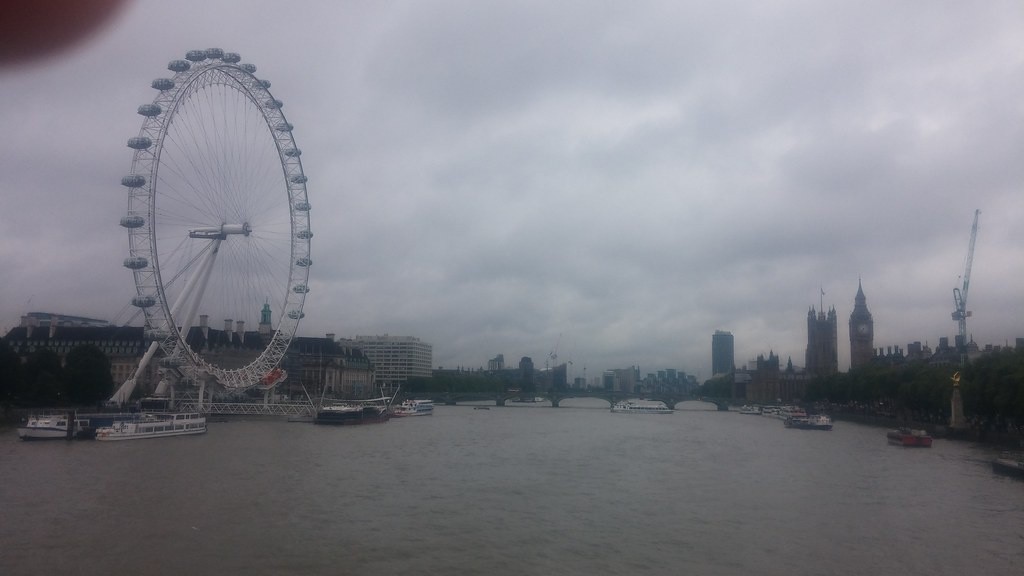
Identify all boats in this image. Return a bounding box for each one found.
[783,414,833,430]
[16,407,91,440]
[392,398,435,417]
[609,401,675,415]
[738,403,808,422]
[886,426,933,448]
[991,453,1024,476]
[95,411,207,441]
[313,404,388,425]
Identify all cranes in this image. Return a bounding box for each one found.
[950,209,984,354]
